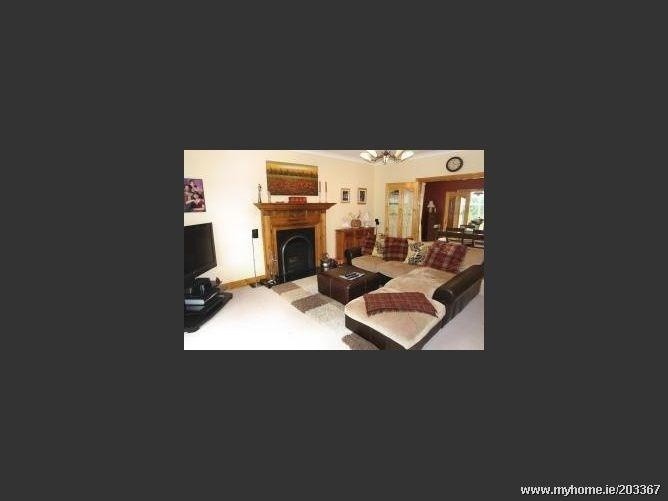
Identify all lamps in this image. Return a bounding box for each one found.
[249,228,262,288]
[360,150,414,164]
[427,201,436,213]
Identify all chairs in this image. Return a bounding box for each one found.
[446,225,476,246]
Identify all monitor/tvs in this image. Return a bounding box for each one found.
[184,222,217,287]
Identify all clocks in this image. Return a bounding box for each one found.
[446,158,463,172]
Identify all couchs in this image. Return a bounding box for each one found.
[344,232,484,350]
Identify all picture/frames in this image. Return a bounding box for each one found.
[357,187,367,204]
[341,188,350,203]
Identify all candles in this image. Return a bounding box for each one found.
[319,181,321,192]
[325,182,327,192]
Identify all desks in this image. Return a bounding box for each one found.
[184,292,233,333]
[435,231,475,247]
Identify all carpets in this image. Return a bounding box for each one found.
[269,275,380,350]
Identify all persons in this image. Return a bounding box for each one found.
[184,180,206,212]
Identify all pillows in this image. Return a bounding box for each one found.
[365,231,464,272]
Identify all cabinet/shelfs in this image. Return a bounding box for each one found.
[385,183,418,241]
[335,226,375,260]
